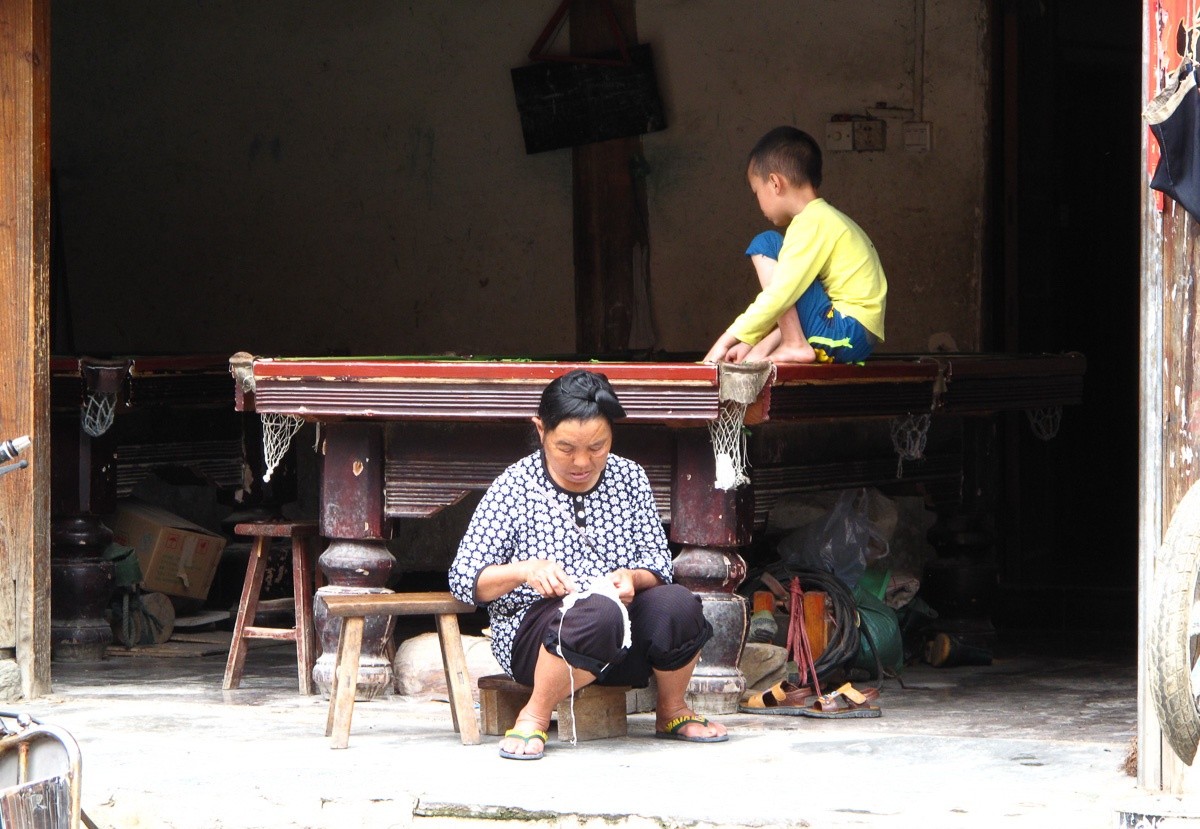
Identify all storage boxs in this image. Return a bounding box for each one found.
[113,498,227,600]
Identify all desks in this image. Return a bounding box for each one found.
[51,344,355,664]
[228,347,1090,716]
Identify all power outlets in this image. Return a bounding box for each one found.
[853,120,885,152]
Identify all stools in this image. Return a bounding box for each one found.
[476,675,632,741]
[222,518,324,695]
[320,593,480,749]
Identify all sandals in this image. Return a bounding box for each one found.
[738,680,811,716]
[802,682,882,718]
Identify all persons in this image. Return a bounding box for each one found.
[448,369,730,761]
[703,126,889,363]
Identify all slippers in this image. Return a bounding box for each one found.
[656,715,729,742]
[499,729,548,760]
